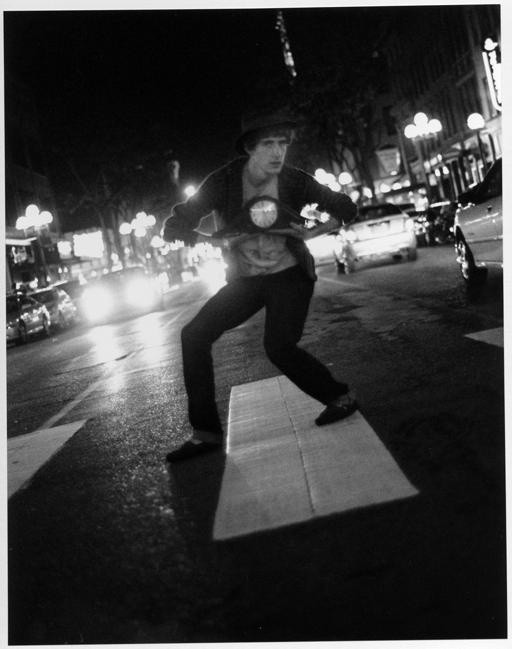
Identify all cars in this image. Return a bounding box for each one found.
[333,198,458,275]
[6,267,163,346]
[454,158,502,285]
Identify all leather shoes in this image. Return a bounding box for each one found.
[315,390,359,426]
[167,435,222,463]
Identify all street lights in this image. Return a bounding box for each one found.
[15,204,53,286]
[404,111,443,202]
[467,112,488,173]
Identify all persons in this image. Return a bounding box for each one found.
[164,103,359,460]
